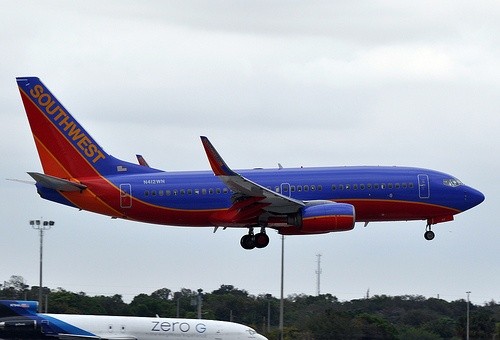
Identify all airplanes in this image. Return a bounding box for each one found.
[0,299,269,340]
[14,75,485,249]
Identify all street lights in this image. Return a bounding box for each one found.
[29,216,56,313]
[191,288,204,319]
